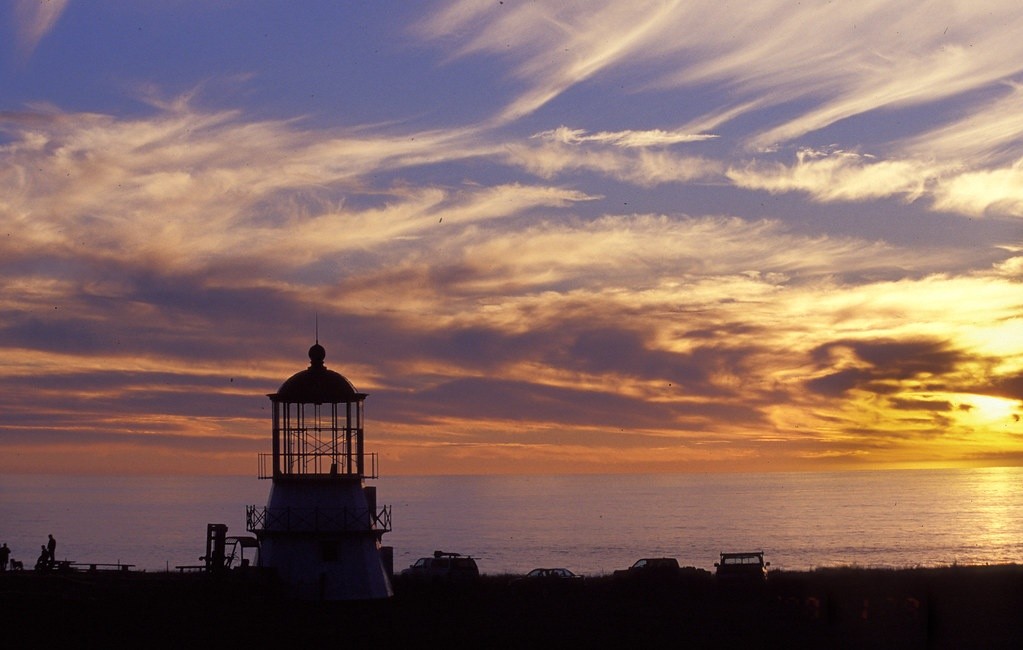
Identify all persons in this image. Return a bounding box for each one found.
[47,535,56,560]
[37,545,49,562]
[0,543,11,569]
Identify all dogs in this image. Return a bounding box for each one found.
[9,558,24,570]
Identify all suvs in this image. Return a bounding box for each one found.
[713,550,771,597]
[400,551,479,589]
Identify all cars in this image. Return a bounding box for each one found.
[526,567,585,589]
[614,557,682,588]
